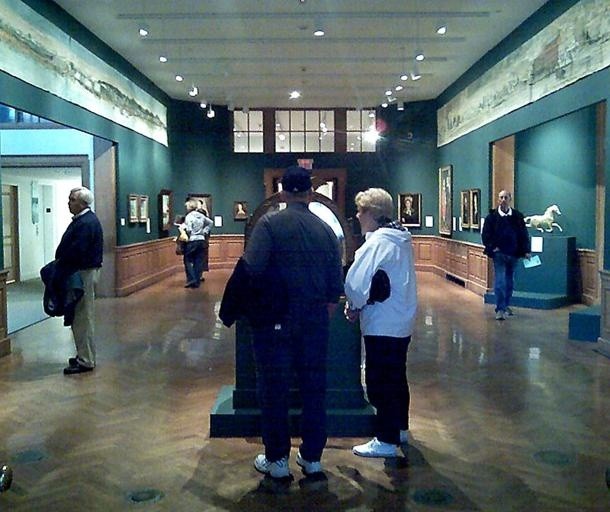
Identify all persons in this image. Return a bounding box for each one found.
[173,199,215,288]
[482,190,531,320]
[219,165,345,479]
[342,187,417,458]
[41,187,103,374]
[472,192,479,224]
[400,197,419,224]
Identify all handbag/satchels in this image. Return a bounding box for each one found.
[176,240,186,255]
[343,261,391,304]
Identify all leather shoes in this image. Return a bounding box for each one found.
[64,365,94,374]
[69,358,78,365]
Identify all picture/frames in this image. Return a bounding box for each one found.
[460,192,469,228]
[469,189,481,229]
[233,200,248,220]
[157,190,174,234]
[187,193,212,221]
[439,165,453,235]
[397,193,422,227]
[139,195,149,222]
[128,194,138,223]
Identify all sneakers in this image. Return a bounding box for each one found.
[353,437,397,457]
[296,452,322,474]
[254,454,290,478]
[505,307,513,315]
[185,279,199,288]
[495,310,506,320]
[283,167,312,192]
[400,430,409,442]
